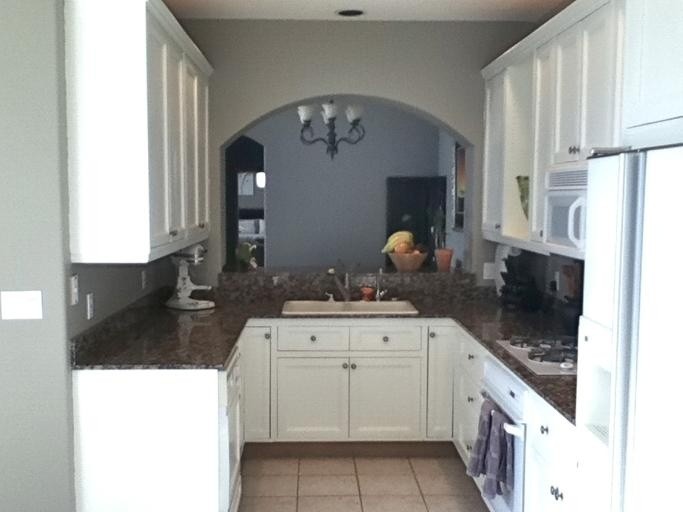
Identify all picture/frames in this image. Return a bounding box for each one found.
[449,136,467,233]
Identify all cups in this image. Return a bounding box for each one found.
[435,248,452,273]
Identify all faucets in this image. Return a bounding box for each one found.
[375,267,398,302]
[325,267,350,301]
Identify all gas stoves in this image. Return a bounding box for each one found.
[496,333,578,375]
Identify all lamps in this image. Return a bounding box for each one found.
[296,97,368,158]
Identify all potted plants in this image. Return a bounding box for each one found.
[430,205,455,271]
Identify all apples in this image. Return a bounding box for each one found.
[394,244,408,253]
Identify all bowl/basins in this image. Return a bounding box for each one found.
[388,250,428,273]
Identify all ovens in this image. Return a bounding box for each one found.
[469,354,527,512]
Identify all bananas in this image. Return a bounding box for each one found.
[378,231,414,254]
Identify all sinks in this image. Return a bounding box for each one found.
[281,299,420,315]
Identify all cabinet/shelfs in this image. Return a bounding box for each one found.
[553,1,682,164]
[454,330,577,512]
[242,325,273,441]
[273,324,429,443]
[426,325,456,441]
[63,0,213,264]
[481,25,553,255]
[71,346,247,511]
